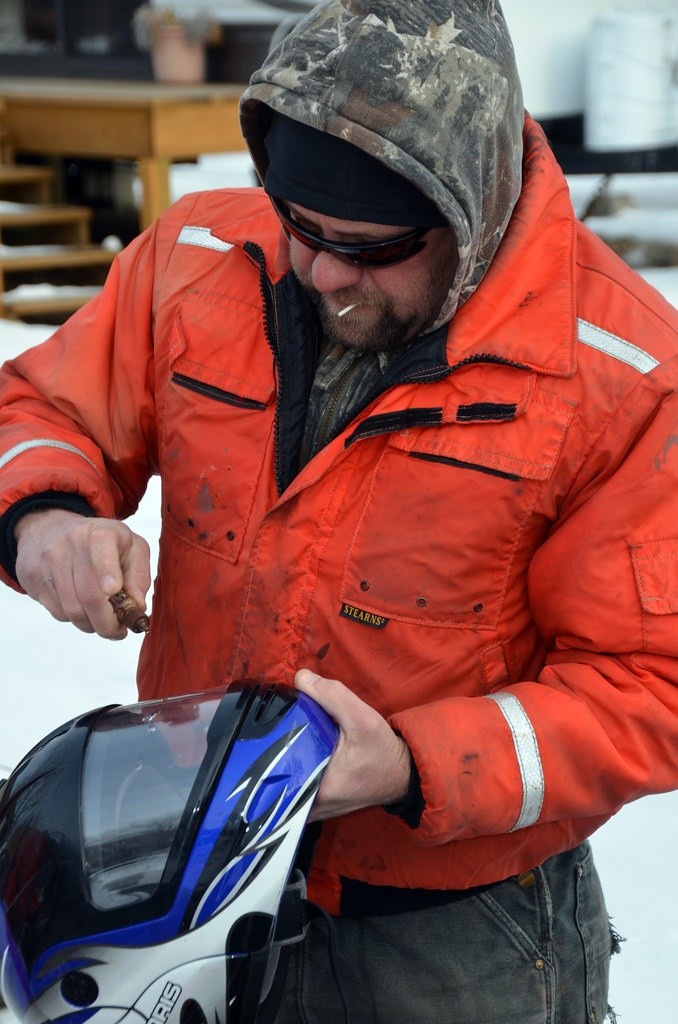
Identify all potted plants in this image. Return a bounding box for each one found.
[130,0,222,84]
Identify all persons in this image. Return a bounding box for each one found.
[0,0,677,1024]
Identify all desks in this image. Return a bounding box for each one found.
[1,76,259,230]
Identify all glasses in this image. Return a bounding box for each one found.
[268,191,431,270]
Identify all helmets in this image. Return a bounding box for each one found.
[0,677,340,1023]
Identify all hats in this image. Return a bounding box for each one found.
[260,108,452,229]
